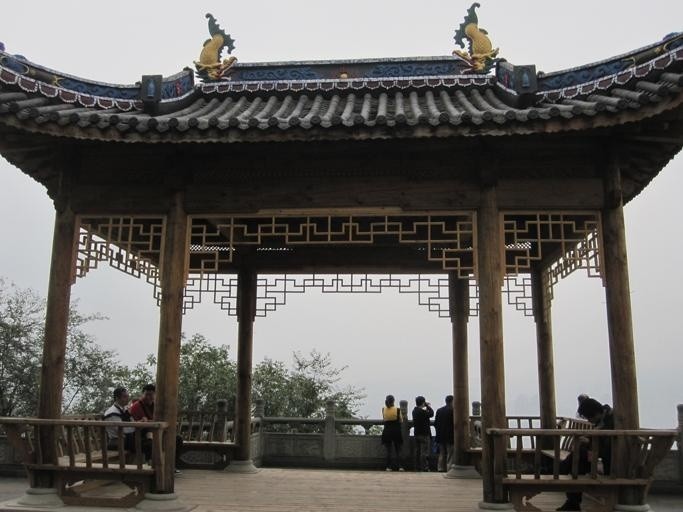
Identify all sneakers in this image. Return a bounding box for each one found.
[557,503,581,511]
[386,467,392,471]
[400,467,410,471]
[425,468,435,472]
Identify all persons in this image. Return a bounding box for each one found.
[381,394,406,471]
[433,395,454,471]
[413,395,434,471]
[556,397,614,511]
[129,386,183,475]
[103,389,154,465]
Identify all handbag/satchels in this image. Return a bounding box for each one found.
[396,429,403,444]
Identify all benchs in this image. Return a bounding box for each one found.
[0,410,233,492]
[465,415,679,510]
